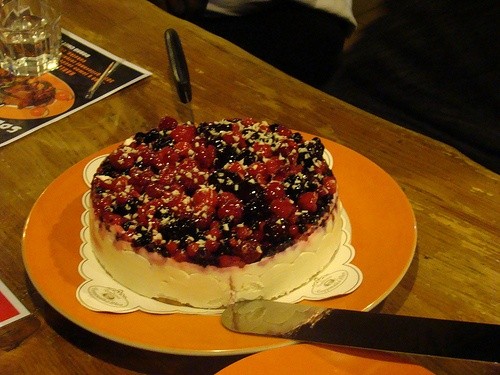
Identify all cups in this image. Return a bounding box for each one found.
[0,0,62,77]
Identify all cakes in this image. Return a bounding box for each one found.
[89,115,342,310]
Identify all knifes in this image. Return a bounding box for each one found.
[221,298,500,363]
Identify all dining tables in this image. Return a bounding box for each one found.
[0,1,498,374]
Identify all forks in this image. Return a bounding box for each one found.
[164,29,195,128]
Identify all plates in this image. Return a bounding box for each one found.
[213,342,436,375]
[20,128,419,357]
